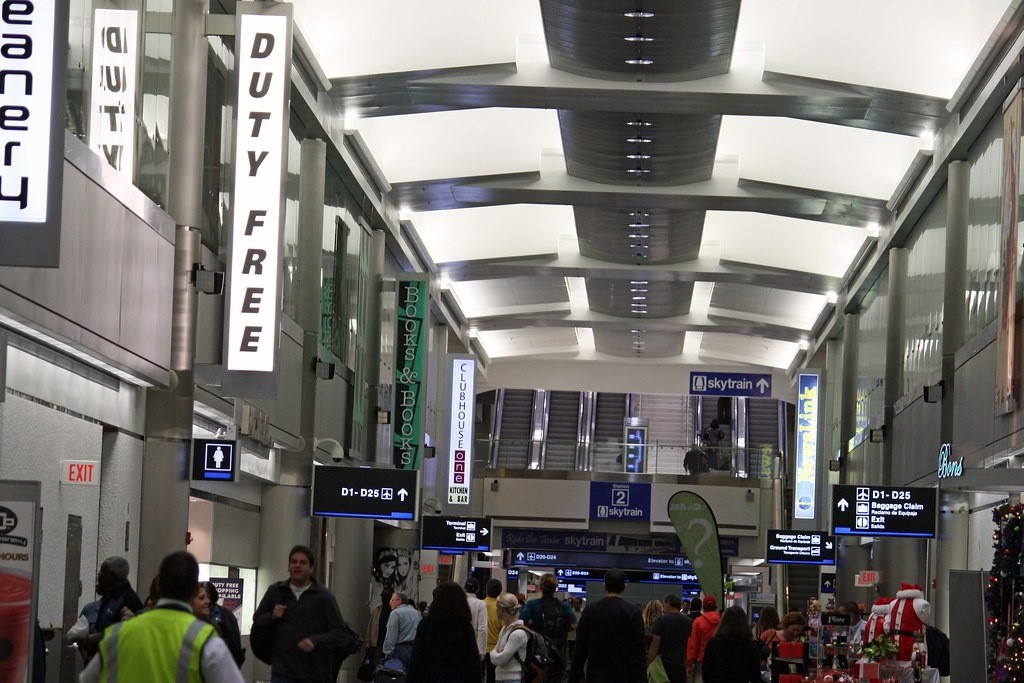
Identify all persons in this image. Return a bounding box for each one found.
[636,599,692,649]
[519,574,578,683]
[570,568,647,683]
[464,577,488,663]
[883,582,933,661]
[561,597,585,683]
[704,419,725,470]
[67,556,144,669]
[253,545,347,683]
[484,578,507,683]
[758,611,808,683]
[383,593,423,683]
[405,598,429,619]
[149,574,247,670]
[79,551,245,683]
[407,581,482,683]
[683,443,706,475]
[366,587,394,683]
[861,596,895,646]
[490,592,530,683]
[702,605,771,683]
[839,601,868,643]
[752,605,780,641]
[647,594,693,683]
[687,595,721,677]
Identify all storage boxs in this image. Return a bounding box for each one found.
[777,640,882,683]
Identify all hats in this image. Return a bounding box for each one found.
[465,577,480,588]
[703,595,716,609]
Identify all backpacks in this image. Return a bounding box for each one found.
[507,624,567,683]
[540,597,570,642]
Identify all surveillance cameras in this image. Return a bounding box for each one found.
[435,502,442,514]
[331,445,344,461]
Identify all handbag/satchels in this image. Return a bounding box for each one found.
[357,654,374,682]
[250,581,280,665]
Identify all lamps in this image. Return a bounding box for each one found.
[923,383,942,403]
[869,428,882,443]
[378,407,390,424]
[424,443,435,458]
[830,459,840,471]
[194,263,225,296]
[315,359,335,380]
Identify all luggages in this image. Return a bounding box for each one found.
[373,657,406,683]
